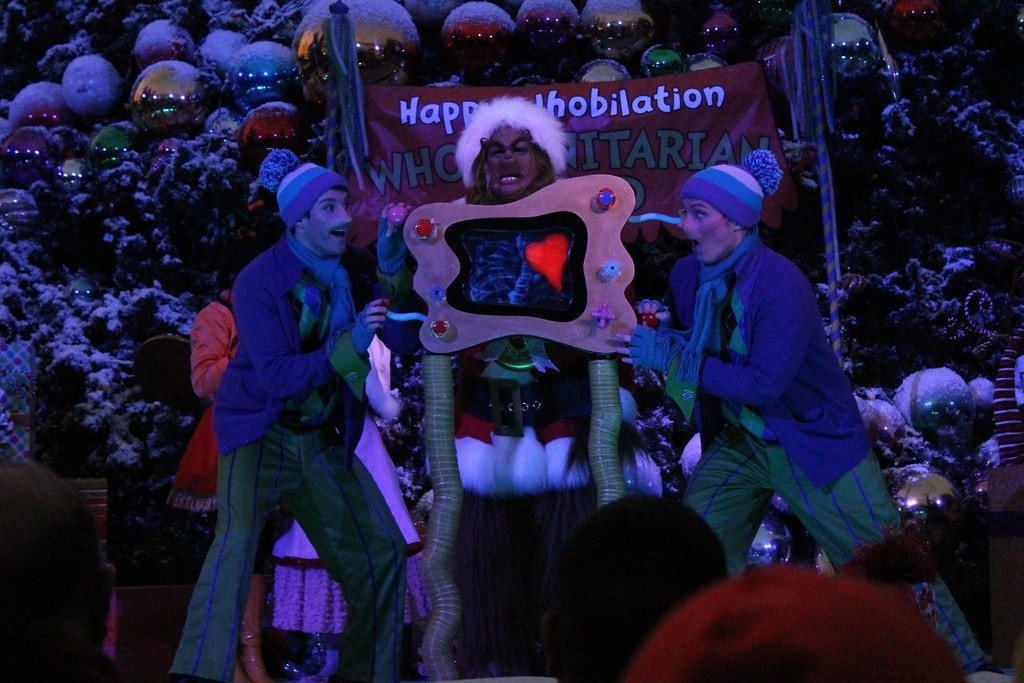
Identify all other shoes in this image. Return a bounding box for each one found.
[969,662,1004,675]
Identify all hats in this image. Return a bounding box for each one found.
[259,149,350,231]
[455,97,567,188]
[680,149,783,229]
[622,567,966,683]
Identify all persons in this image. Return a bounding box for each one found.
[544,491,729,683]
[372,101,673,682]
[614,149,1005,675]
[163,152,409,683]
[0,465,120,682]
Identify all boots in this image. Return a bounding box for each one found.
[232,574,275,683]
